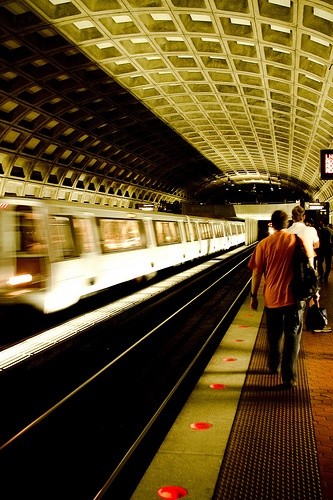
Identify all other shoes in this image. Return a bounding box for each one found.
[286,382,296,387]
[268,368,279,375]
[319,274,328,283]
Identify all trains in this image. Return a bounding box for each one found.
[0,198,248,321]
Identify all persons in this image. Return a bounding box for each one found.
[249,210,316,385]
[283,211,333,283]
[287,205,332,333]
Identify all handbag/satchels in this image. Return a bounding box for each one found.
[291,235,317,301]
[303,296,328,331]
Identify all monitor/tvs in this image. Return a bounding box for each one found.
[321,149,333,180]
